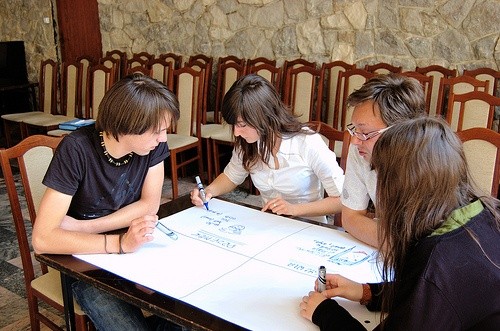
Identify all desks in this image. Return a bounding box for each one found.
[33,188,394,331]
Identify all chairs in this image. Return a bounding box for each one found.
[0,49,500,331]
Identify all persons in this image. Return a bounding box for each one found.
[32,75,181,331]
[188,74,346,224]
[341,74,429,251]
[299,117,500,331]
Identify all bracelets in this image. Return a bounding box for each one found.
[117,233,127,254]
[104,234,112,253]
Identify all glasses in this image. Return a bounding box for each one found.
[346,124,397,141]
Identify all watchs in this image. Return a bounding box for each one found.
[360,284,371,306]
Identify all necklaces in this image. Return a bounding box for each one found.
[98,129,134,167]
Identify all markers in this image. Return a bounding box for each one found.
[195,176,209,210]
[318,266,326,293]
[155,221,178,240]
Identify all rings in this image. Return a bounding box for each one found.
[274,203,277,207]
